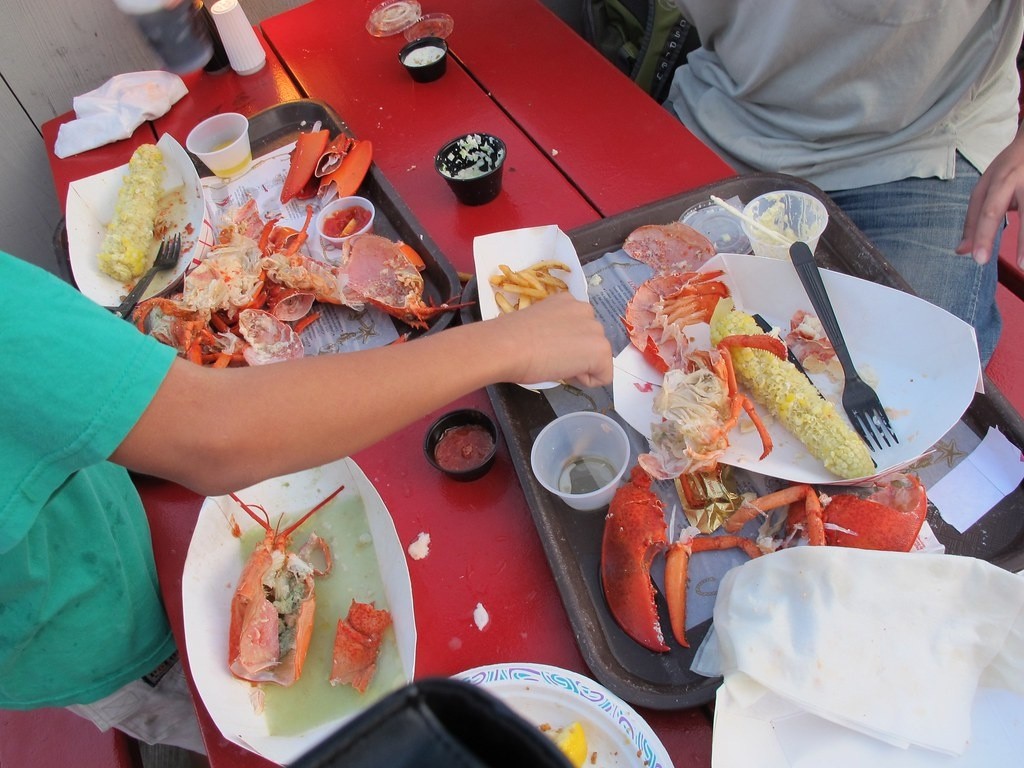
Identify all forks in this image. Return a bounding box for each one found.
[113,230,182,319]
[785,241,899,453]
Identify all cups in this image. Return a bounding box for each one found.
[113,0,215,77]
[317,196,376,250]
[740,189,829,266]
[423,407,498,481]
[184,112,252,180]
[395,37,449,82]
[530,411,630,511]
[432,133,507,206]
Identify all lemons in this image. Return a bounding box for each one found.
[554,720,587,768]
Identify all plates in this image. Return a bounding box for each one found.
[443,662,676,768]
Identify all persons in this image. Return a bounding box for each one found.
[0,255,615,755]
[665,0,1024,369]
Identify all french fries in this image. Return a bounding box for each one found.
[491,260,570,315]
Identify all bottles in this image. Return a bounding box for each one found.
[210,0,267,76]
[179,1,232,74]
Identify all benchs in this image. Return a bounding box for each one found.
[0,709,141,768]
[985,199,1023,438]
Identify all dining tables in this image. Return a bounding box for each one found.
[41,2,740,768]
